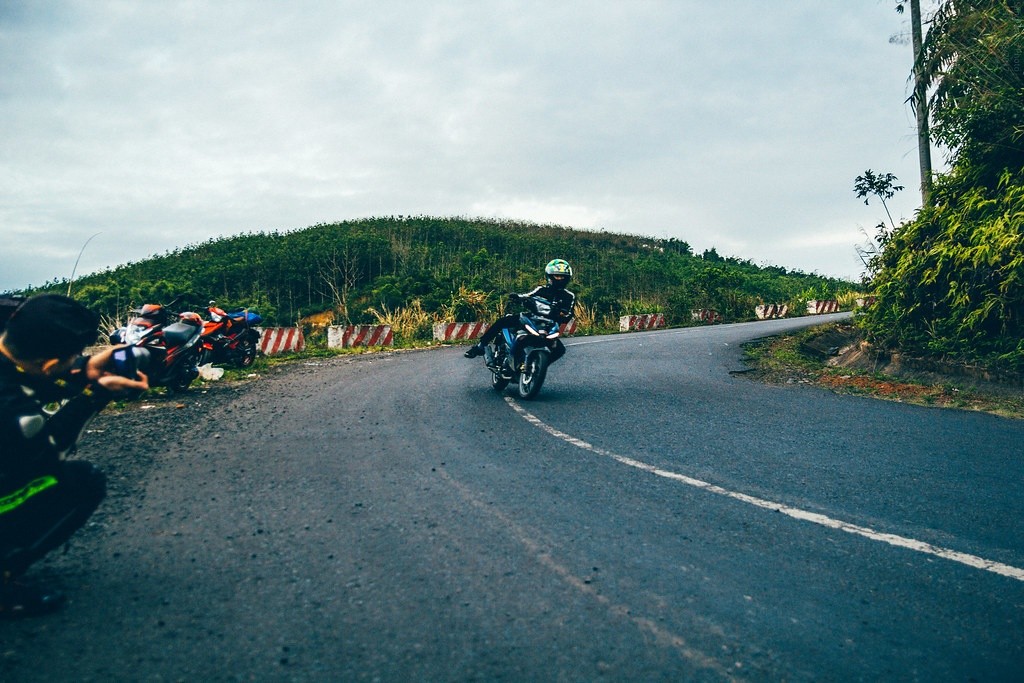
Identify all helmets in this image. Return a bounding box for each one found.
[545,259,573,290]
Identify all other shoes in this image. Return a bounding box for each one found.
[0,568,70,617]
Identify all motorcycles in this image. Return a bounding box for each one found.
[110,298,263,395]
[483,294,569,400]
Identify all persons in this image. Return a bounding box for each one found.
[0,294,150,617]
[464,258,576,359]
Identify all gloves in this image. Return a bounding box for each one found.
[509,293,519,303]
[561,317,571,323]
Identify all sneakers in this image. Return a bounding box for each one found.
[464,345,485,358]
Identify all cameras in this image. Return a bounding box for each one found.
[112,345,152,381]
